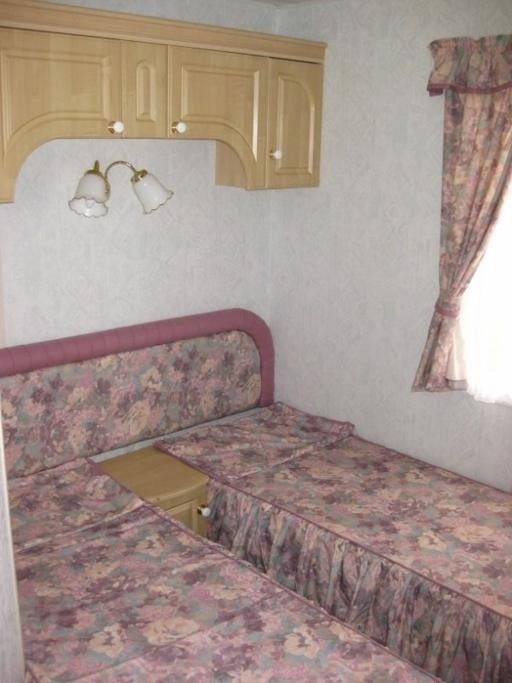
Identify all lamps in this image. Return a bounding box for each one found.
[67,161,173,220]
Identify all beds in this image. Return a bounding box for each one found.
[155,310,512,682]
[0,322,449,683]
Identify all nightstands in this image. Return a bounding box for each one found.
[93,446,212,540]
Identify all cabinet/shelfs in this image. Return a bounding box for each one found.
[0,0,327,203]
